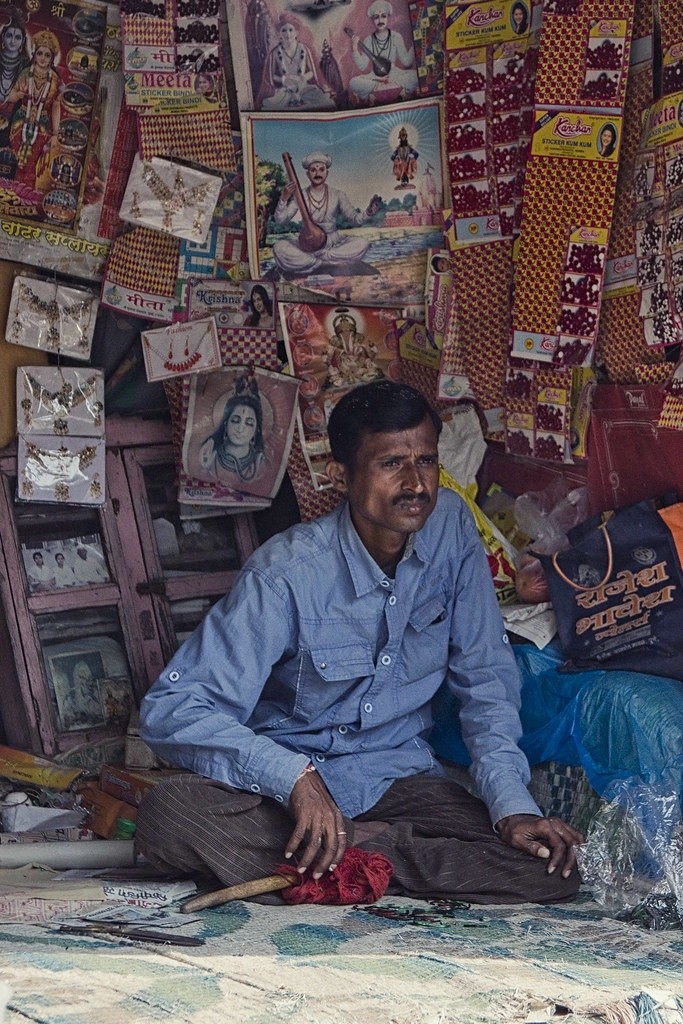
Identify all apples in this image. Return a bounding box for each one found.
[517,544,550,604]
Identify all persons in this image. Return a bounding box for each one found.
[122,690,133,715]
[27,551,55,592]
[598,124,617,157]
[244,285,273,329]
[431,255,451,273]
[511,3,528,34]
[52,552,77,588]
[133,381,584,904]
[73,547,108,584]
[106,689,119,718]
[196,76,212,95]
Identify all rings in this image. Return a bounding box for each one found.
[337,832,347,835]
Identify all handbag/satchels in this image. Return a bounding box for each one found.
[526,495,683,681]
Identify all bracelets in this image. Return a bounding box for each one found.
[298,766,315,779]
